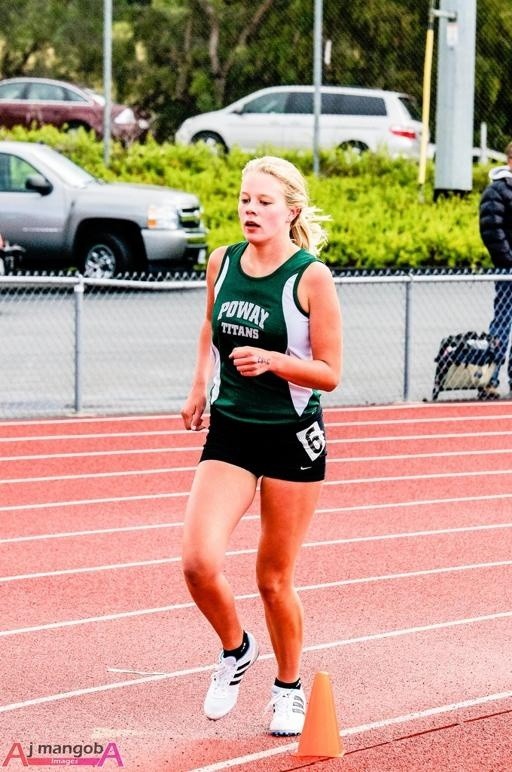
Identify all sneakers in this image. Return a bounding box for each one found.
[205,630,258,722]
[268,684,305,738]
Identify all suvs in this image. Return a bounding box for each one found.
[170,80,436,166]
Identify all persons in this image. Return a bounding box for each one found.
[479,141,512,398]
[182,156,342,735]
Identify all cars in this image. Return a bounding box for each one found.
[0,138,214,292]
[0,72,149,143]
